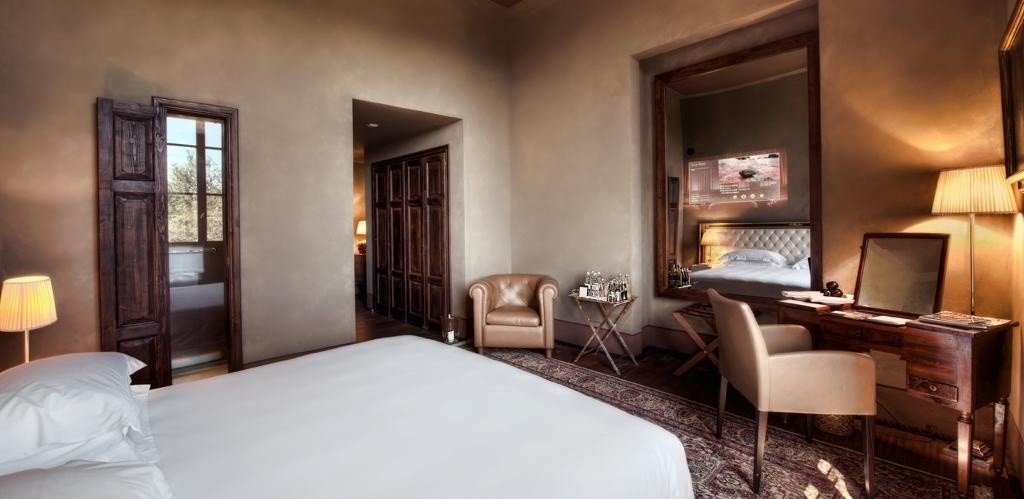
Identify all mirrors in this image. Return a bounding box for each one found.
[654,30,821,313]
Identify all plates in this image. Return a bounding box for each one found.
[812,296,856,306]
[781,290,821,301]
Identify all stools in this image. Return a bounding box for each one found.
[673,302,762,377]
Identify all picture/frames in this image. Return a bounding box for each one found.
[851,232,950,320]
[998,1,1024,186]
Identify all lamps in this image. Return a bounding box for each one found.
[0,274,58,363]
[932,164,1017,317]
[700,231,719,263]
[356,220,367,254]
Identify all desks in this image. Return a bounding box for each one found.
[678,285,692,291]
[568,292,639,376]
[355,254,366,304]
[776,299,1019,499]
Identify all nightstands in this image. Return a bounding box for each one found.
[692,264,713,271]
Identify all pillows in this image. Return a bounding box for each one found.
[719,249,809,270]
[0,351,173,499]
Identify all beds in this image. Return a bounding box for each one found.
[0,333,696,499]
[690,222,811,298]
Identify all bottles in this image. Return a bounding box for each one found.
[579,271,632,302]
[445,312,457,345]
[667,263,691,287]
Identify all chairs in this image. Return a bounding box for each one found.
[471,273,558,359]
[705,285,877,499]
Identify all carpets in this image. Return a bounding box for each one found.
[458,346,1011,499]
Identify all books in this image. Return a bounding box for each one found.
[779,288,854,311]
[920,310,1012,330]
